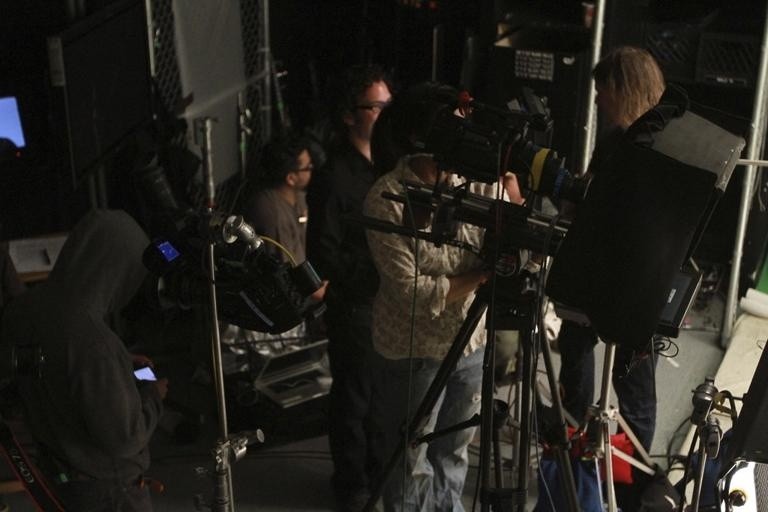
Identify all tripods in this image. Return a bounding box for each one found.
[361,325,589,512]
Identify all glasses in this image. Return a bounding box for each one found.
[353,103,382,113]
[298,164,314,172]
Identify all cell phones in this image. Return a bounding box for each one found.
[131,365,158,386]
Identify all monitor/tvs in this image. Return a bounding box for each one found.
[0,96,31,155]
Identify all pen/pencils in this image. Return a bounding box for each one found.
[44,248,50,264]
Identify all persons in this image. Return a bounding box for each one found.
[481,163,541,382]
[293,98,339,178]
[304,69,392,512]
[243,134,330,301]
[542,48,688,510]
[1,208,171,512]
[359,77,534,512]
